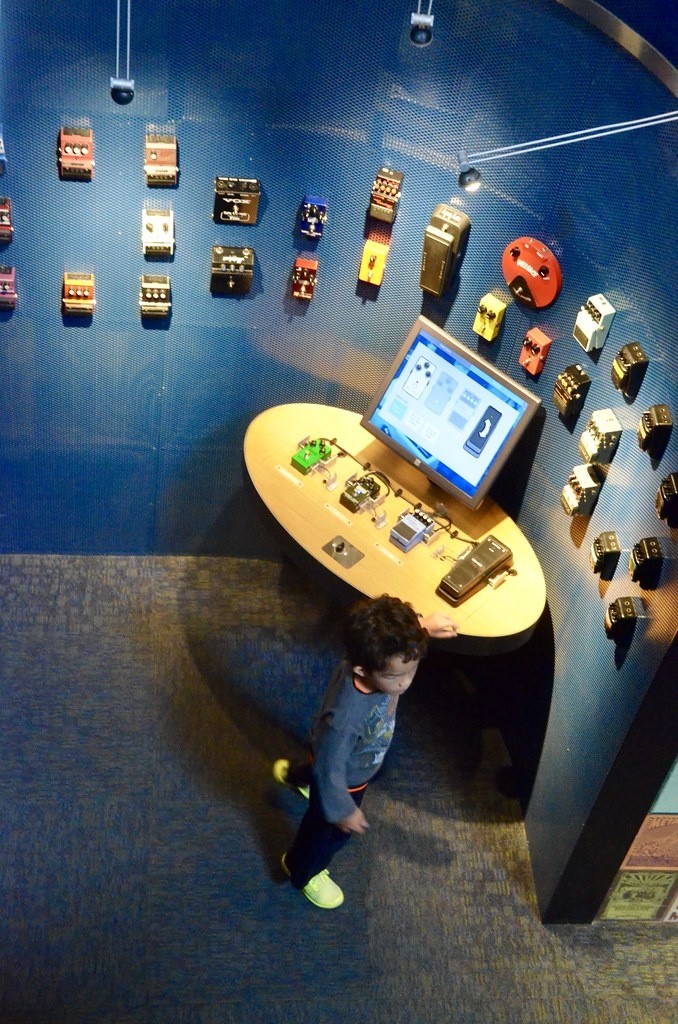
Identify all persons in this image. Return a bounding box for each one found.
[273,593,459,909]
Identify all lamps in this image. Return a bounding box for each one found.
[409,0,436,49]
[457,111,678,193]
[108,0,136,106]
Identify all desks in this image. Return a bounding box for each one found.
[241,402,549,655]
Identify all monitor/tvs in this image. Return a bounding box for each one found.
[359,315,542,511]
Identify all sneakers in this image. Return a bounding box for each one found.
[273,759,310,799]
[281,852,345,908]
